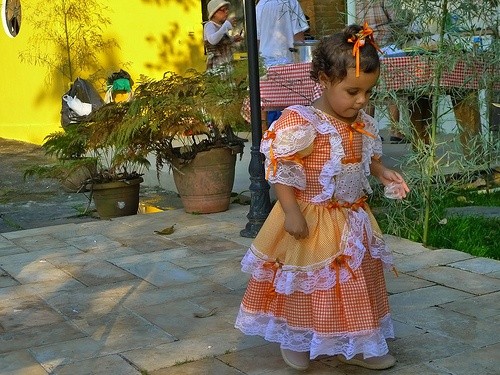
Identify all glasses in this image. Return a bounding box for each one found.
[219,7,229,12]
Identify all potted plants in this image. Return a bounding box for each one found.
[25,100,143,219]
[130,57,262,215]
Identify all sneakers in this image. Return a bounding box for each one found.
[338,350,395,370]
[279,344,310,370]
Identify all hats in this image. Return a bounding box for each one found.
[206,0,231,22]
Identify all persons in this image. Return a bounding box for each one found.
[251,0,310,129]
[233,25,412,370]
[201,0,244,133]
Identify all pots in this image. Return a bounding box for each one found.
[290,38,325,64]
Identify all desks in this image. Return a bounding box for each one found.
[254,49,500,166]
